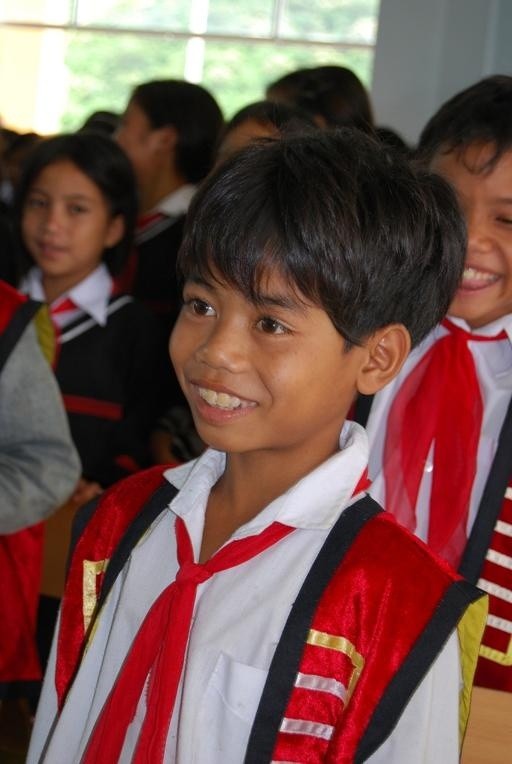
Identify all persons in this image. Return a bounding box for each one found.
[0,67,416,509]
[21,130,464,763]
[351,76,512,692]
[1,278,84,763]
